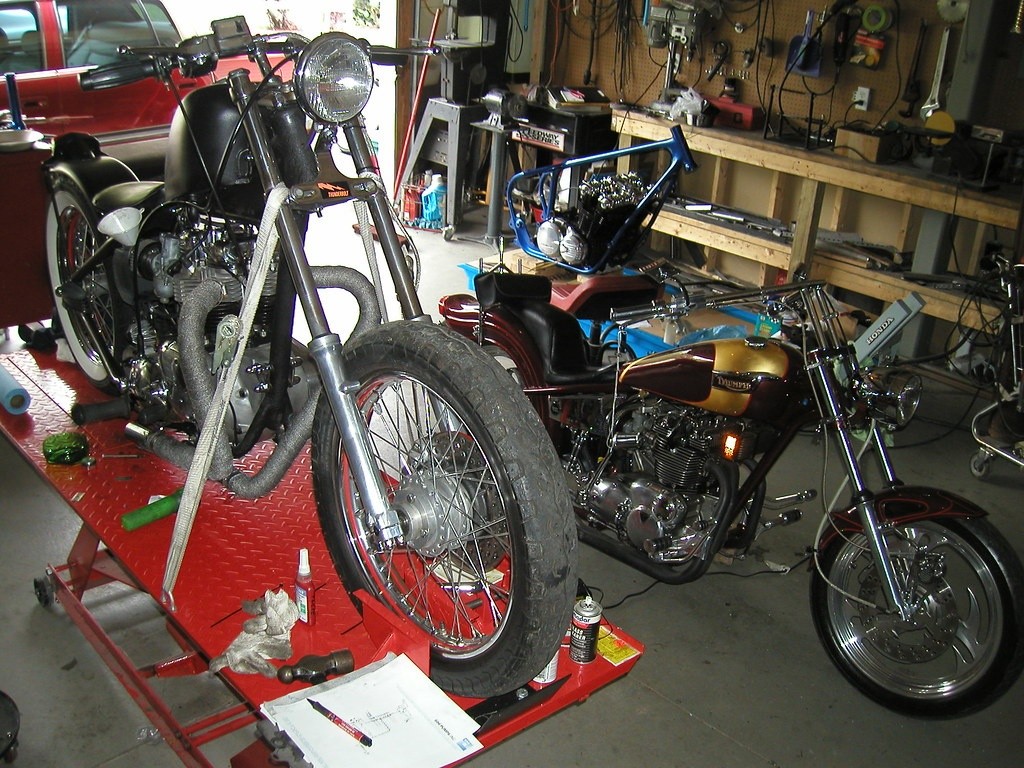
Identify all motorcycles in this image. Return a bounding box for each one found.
[41,14,576,695]
[438,243,1024,723]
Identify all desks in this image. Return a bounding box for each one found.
[390,100,530,226]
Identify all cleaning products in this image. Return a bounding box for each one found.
[421,174,447,221]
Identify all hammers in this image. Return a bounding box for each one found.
[277,647,355,686]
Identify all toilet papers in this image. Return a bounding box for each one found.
[0,362,31,415]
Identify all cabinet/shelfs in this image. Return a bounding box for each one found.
[610,103,1024,402]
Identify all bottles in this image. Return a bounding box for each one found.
[568,595,603,665]
[295,549,316,627]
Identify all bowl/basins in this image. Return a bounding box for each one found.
[43,431,89,465]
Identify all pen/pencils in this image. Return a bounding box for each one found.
[306,698,372,747]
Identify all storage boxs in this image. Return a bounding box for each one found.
[833,123,897,164]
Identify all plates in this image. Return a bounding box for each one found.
[0,129,44,152]
